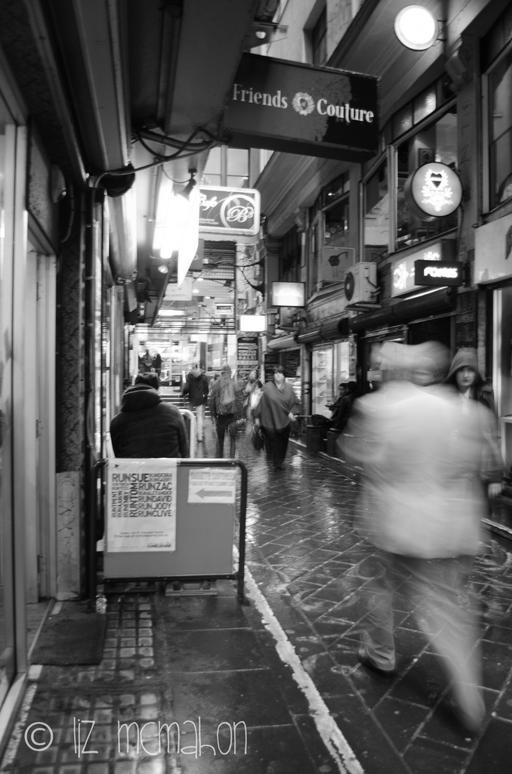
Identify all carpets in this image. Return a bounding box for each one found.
[27,609,107,666]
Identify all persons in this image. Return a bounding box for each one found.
[109,371,190,458]
[178,362,305,472]
[444,347,504,516]
[138,348,165,382]
[337,340,510,735]
[311,379,374,438]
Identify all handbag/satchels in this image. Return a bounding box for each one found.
[251,425,263,451]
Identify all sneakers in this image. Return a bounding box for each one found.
[440,699,478,736]
[357,648,395,673]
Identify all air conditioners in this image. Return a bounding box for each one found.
[341,262,379,308]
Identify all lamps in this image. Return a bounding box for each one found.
[178,167,197,200]
